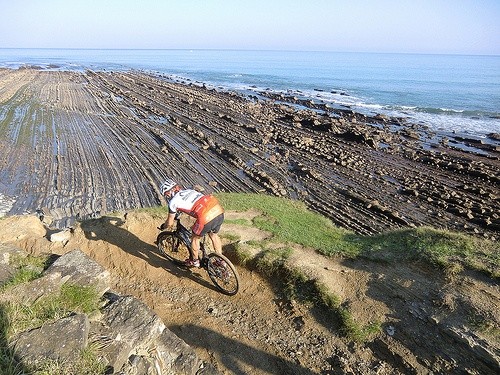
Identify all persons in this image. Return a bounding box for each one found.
[160,179,226,269]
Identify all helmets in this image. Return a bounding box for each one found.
[160,179,177,196]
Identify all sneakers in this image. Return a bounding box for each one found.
[212,258,225,267]
[185,257,200,267]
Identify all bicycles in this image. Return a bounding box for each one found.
[154,212,241,296]
